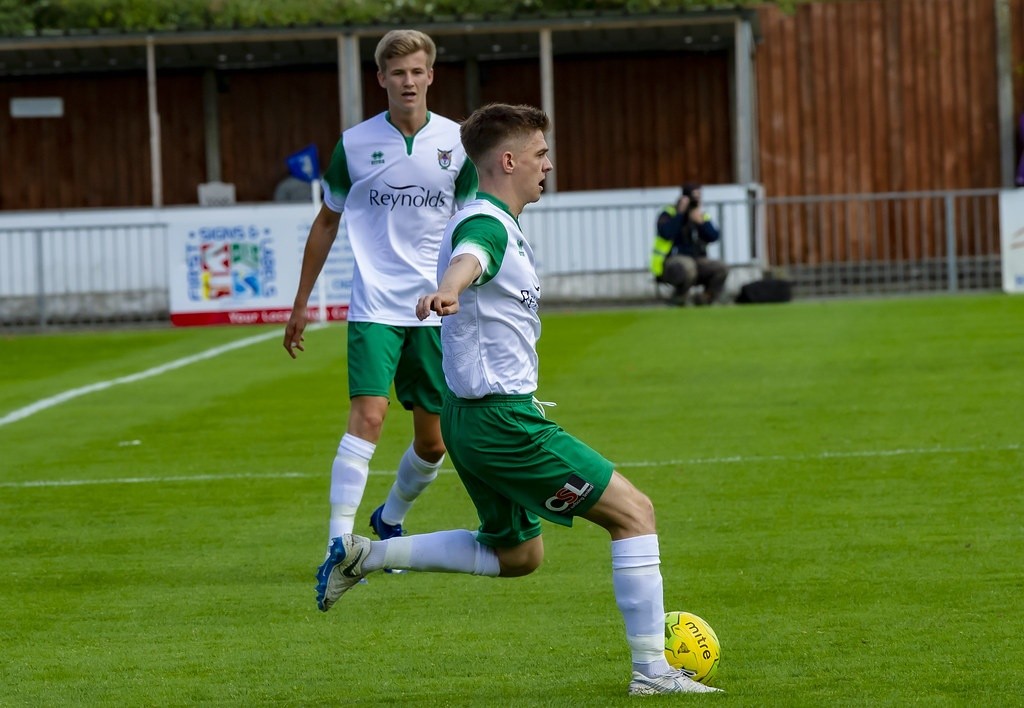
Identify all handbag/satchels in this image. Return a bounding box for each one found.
[738,280,789,303]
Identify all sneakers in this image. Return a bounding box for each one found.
[628,667,724,694]
[370,504,405,576]
[314,534,370,613]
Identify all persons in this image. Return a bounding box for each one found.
[285,31,479,583]
[314,103,725,696]
[650,182,728,307]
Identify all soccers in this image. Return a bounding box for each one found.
[662,611,721,686]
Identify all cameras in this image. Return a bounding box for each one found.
[686,196,698,210]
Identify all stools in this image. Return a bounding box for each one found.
[653,274,682,301]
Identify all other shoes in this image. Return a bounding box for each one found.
[694,293,710,306]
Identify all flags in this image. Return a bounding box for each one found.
[287,145,319,184]
